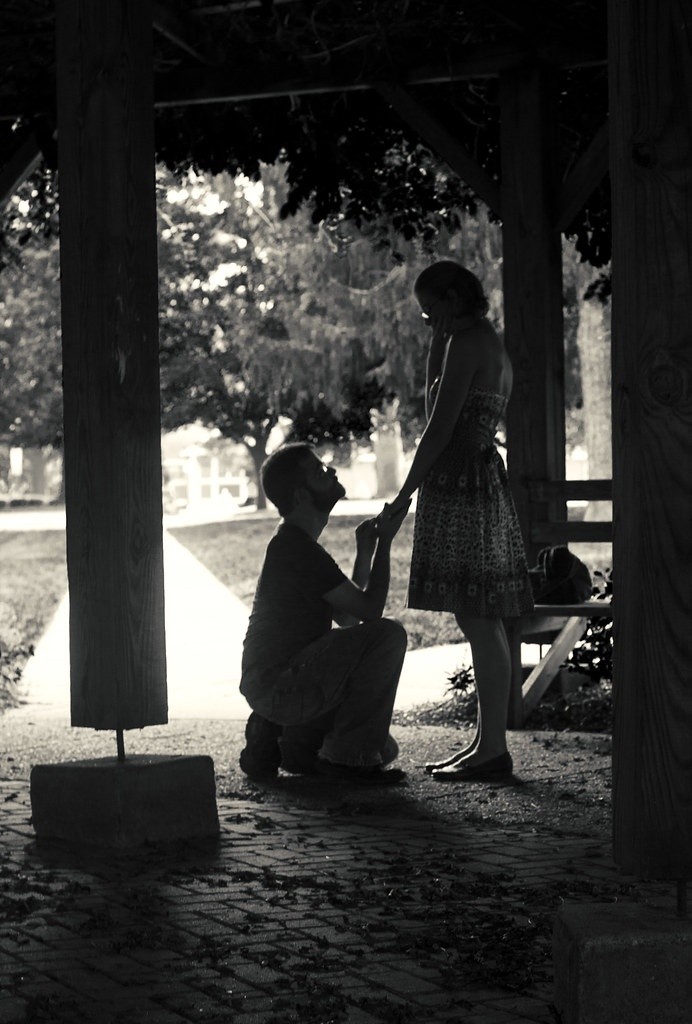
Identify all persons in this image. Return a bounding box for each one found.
[376,262,535,782]
[241,444,411,786]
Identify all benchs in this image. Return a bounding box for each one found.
[503,603,612,731]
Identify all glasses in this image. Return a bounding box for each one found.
[420,288,446,320]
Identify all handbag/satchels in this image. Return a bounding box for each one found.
[527,544,591,605]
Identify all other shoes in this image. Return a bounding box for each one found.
[238,712,284,779]
[314,757,405,786]
[432,753,512,780]
[426,757,459,775]
